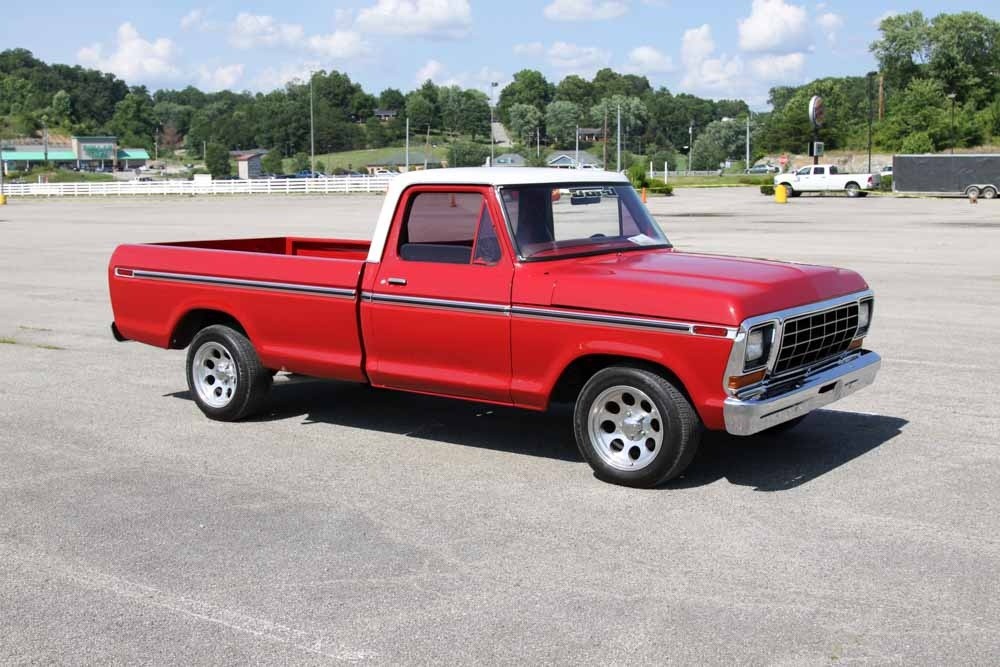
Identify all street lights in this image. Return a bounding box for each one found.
[721,117,749,169]
[153,143,157,160]
[867,72,877,173]
[491,81,498,167]
[948,94,957,154]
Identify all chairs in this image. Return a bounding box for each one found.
[520,222,552,252]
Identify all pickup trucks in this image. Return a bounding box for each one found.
[773,164,881,198]
[108,166,881,488]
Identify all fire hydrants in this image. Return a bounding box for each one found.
[640,188,648,203]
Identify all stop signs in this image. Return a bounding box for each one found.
[779,155,788,164]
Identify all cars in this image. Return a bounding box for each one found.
[275,169,400,178]
[880,165,892,177]
[744,160,779,174]
